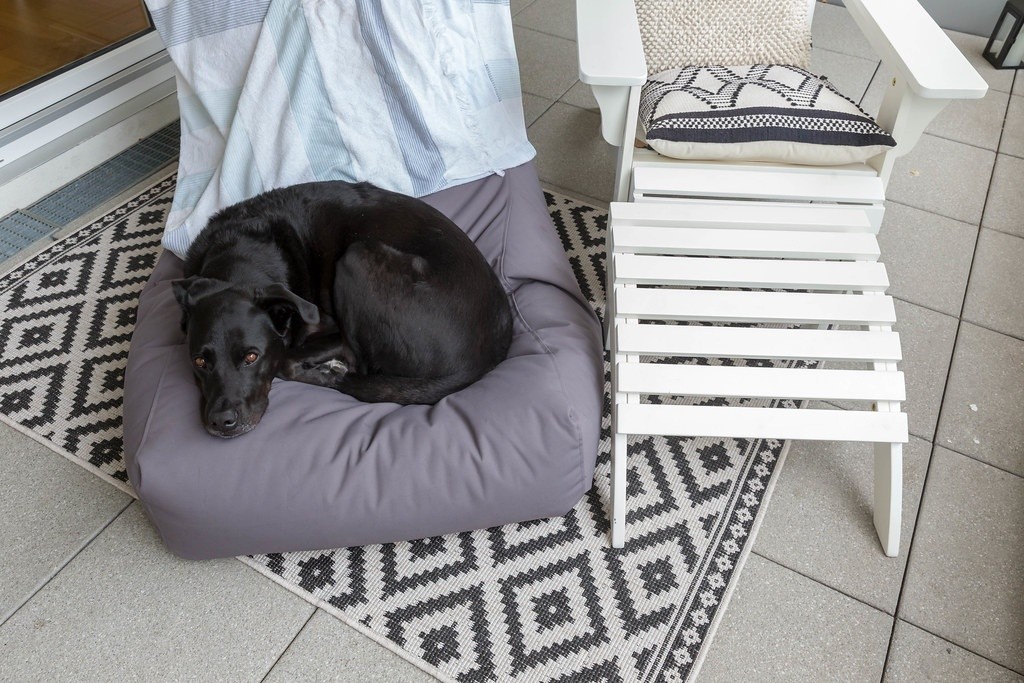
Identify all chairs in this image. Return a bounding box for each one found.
[565,1,993,556]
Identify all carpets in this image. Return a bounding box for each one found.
[4,153,856,682]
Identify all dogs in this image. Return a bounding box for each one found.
[170,181,513,440]
